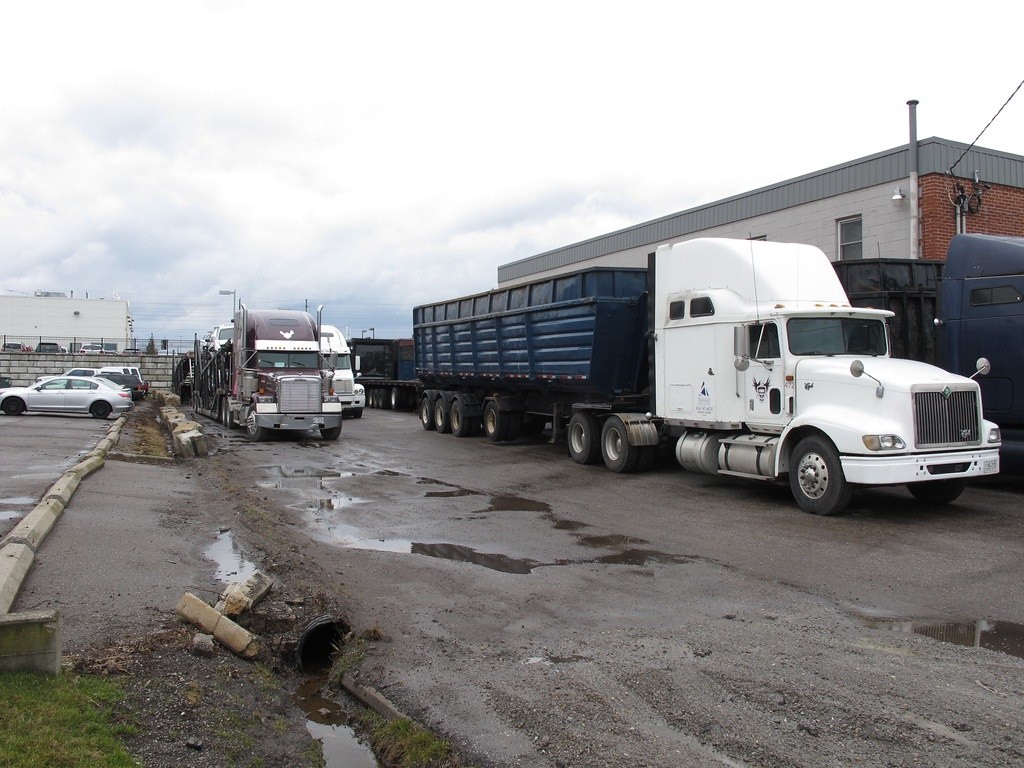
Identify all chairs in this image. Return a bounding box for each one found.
[65,383,73,389]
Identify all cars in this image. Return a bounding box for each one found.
[35,366,149,400]
[0,375,136,420]
[199,324,235,356]
[0,341,27,352]
[76,343,107,355]
[157,350,179,356]
[122,348,144,357]
[34,341,64,354]
[0,375,133,401]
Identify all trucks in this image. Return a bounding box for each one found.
[826,232,1024,484]
[316,324,366,419]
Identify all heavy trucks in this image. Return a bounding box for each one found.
[346,336,424,411]
[191,303,343,443]
[409,237,1003,518]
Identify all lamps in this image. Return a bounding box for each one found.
[127,316,134,332]
[891,185,907,201]
[74,311,79,314]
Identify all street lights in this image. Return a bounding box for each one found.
[218,288,237,319]
[361,329,367,338]
[368,327,375,339]
